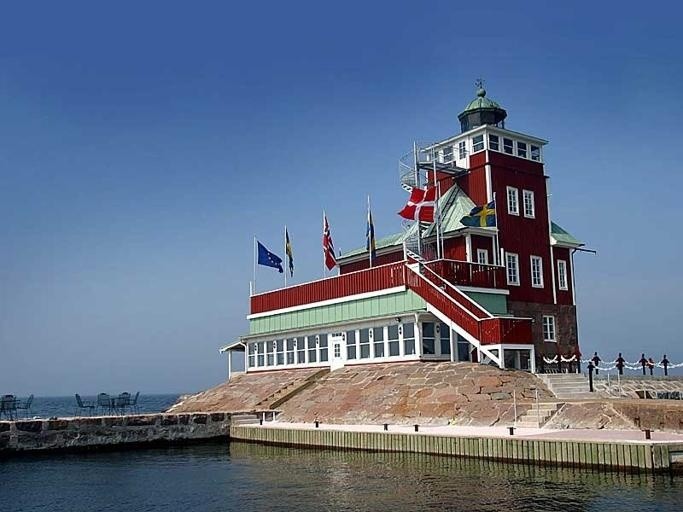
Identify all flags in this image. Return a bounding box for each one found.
[397,184,436,225]
[285,232,294,277]
[364,204,377,259]
[458,201,497,227]
[258,241,282,272]
[321,215,336,271]
